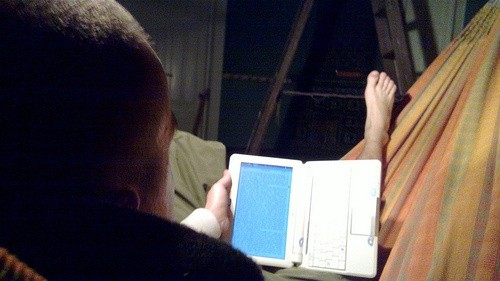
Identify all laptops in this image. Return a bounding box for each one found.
[222,153,382,278]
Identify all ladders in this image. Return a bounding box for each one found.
[246,0,410,157]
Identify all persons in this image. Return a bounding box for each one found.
[2,1,398,281]
